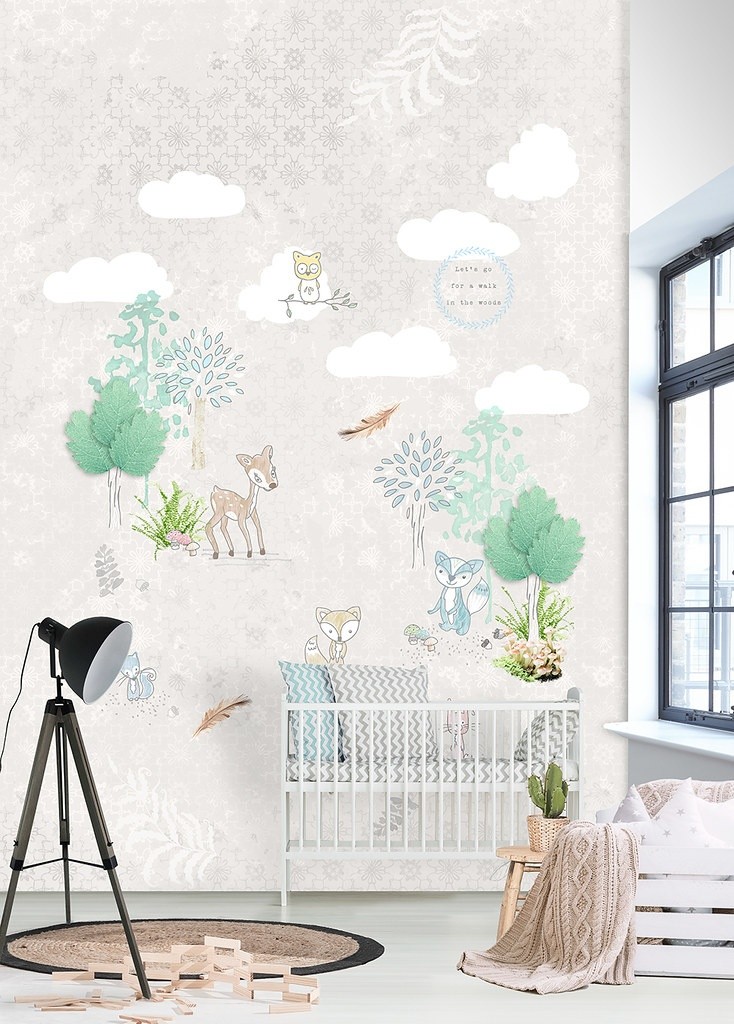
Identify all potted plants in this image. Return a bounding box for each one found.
[527,764,569,853]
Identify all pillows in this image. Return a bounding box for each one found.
[512,700,580,767]
[324,665,442,761]
[276,658,344,763]
[614,777,734,914]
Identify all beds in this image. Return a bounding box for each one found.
[279,687,581,907]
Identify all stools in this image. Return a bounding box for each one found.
[496,846,548,944]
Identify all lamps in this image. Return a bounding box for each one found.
[1,616,153,1001]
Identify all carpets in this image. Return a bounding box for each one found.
[0,918,384,982]
